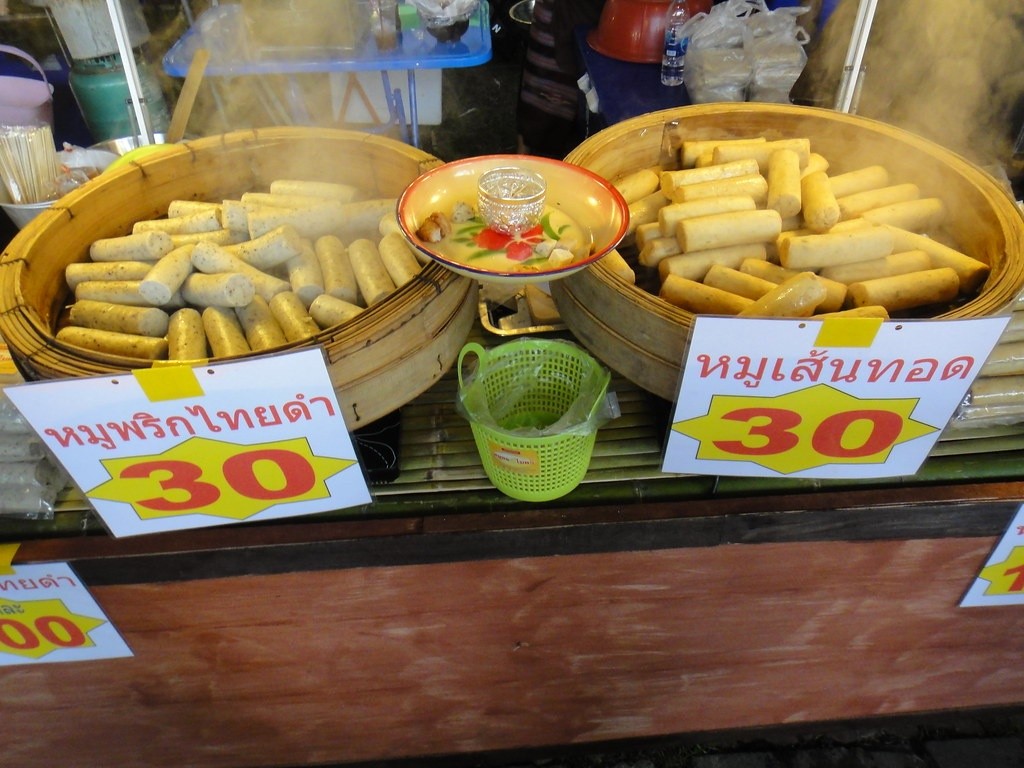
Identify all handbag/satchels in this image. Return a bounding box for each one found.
[676,1,810,105]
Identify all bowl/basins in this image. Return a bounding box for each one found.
[587,1,712,62]
[422,15,470,43]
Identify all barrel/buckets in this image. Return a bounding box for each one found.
[0,45,54,136]
[0,149,126,230]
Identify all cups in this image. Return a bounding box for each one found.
[475,165,546,236]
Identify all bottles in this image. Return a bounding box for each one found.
[662,1,685,86]
[368,0,402,48]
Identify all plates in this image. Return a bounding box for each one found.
[397,154,630,285]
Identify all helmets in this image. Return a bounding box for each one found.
[586,0,713,63]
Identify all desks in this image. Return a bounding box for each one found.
[160,0,492,150]
[575,26,691,128]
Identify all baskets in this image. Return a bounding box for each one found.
[457,339,611,502]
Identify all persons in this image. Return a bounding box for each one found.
[519,0,588,161]
